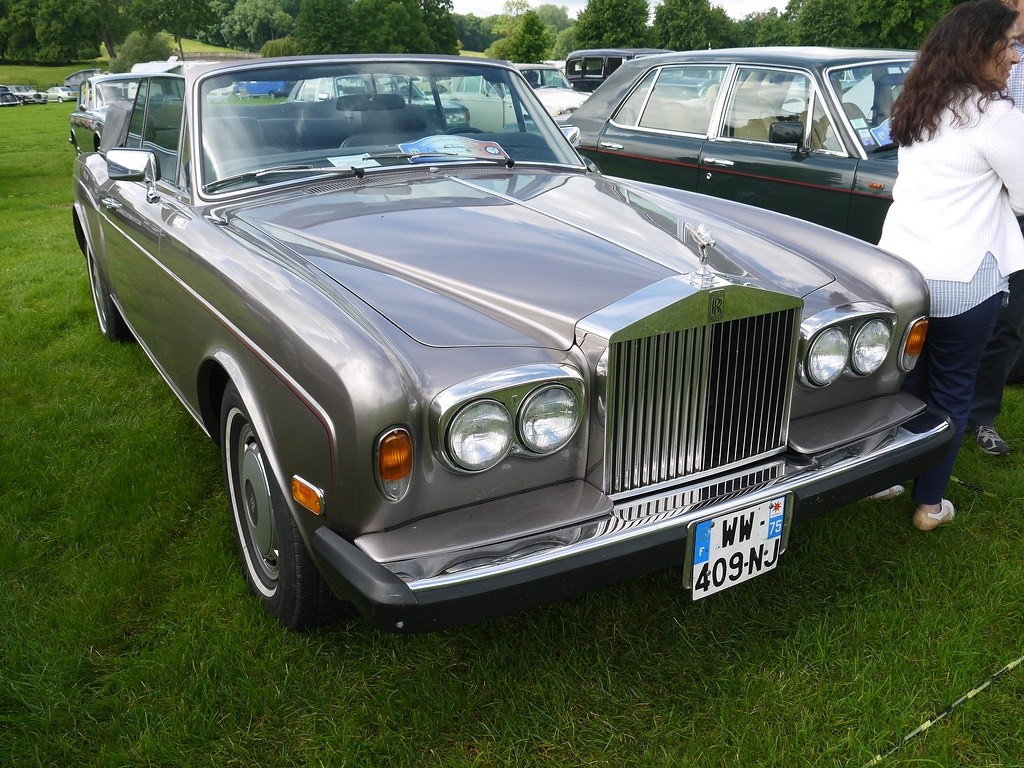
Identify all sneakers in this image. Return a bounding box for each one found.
[965,424,1012,457]
[868,485,906,500]
[912,497,955,531]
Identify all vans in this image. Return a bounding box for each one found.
[565,45,677,94]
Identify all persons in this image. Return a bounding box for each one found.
[966,0,1024,455]
[866,0,1024,531]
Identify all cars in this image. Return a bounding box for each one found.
[71,52,959,633]
[557,45,924,245]
[73,60,602,150]
[0,84,77,105]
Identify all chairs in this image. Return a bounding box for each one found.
[733,88,774,142]
[798,77,870,153]
[338,104,437,147]
[204,117,284,164]
